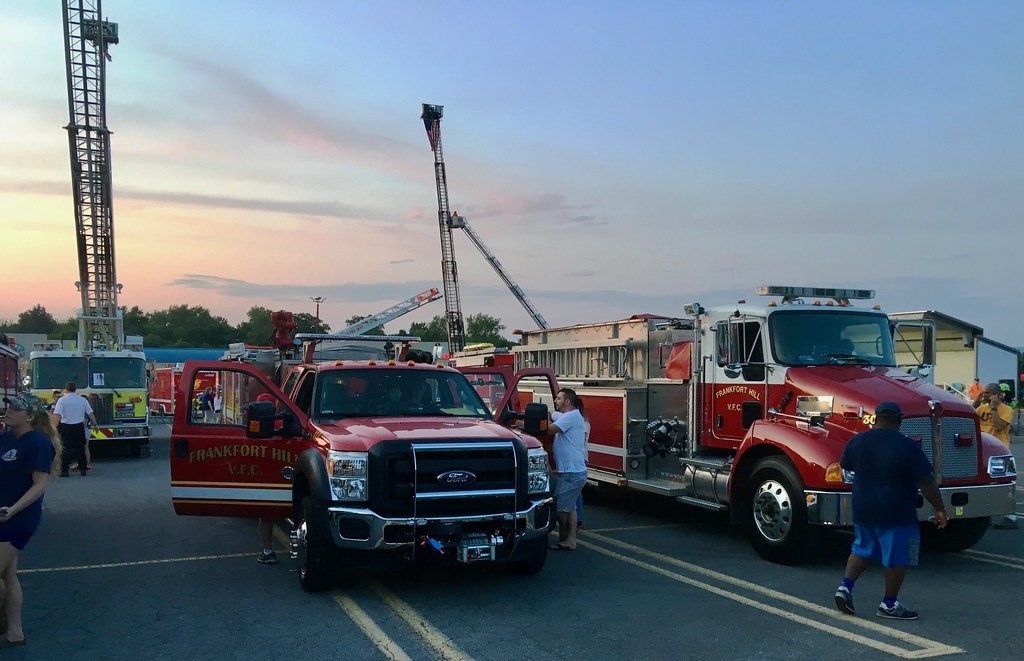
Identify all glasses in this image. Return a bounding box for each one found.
[416,386,425,391]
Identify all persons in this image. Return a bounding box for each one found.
[256,394,282,562]
[968,377,1018,530]
[395,373,444,413]
[0,391,63,648]
[200,385,222,423]
[45,382,100,478]
[833,401,949,619]
[514,389,590,551]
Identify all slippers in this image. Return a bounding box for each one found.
[0,622,26,647]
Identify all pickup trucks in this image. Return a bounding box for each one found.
[169,331,561,594]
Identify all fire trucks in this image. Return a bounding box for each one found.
[422,103,521,424]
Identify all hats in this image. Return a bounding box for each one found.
[2,392,45,415]
[999,383,1010,391]
[257,393,276,402]
[875,401,904,416]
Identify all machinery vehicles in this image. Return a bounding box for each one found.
[23,0,156,459]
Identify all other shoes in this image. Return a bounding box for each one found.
[71,464,79,470]
[576,521,583,531]
[57,472,69,477]
[87,465,92,471]
[81,471,87,476]
[550,539,576,550]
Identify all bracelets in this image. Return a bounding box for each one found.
[93,423,97,427]
[990,407,997,412]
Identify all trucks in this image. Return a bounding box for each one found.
[0,329,19,433]
[485,282,1018,565]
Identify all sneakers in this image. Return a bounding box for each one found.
[258,549,278,562]
[835,581,856,615]
[876,600,918,619]
[994,516,1019,529]
